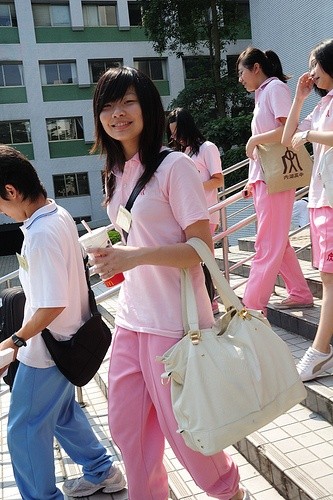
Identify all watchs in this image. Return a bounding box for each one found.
[11,334,27,347]
[302,130,310,142]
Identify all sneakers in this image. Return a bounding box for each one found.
[295,348,333,381]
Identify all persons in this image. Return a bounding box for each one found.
[164,107,225,315]
[0,144,126,500]
[86,65,250,500]
[235,47,315,319]
[280,40,333,382]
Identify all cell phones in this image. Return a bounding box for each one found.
[242,189,249,197]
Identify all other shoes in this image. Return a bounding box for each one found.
[274,293,312,309]
[62,468,125,497]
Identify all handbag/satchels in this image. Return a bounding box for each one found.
[152,236,307,458]
[257,136,317,194]
[27,266,112,387]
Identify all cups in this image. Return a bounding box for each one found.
[78,227,126,287]
